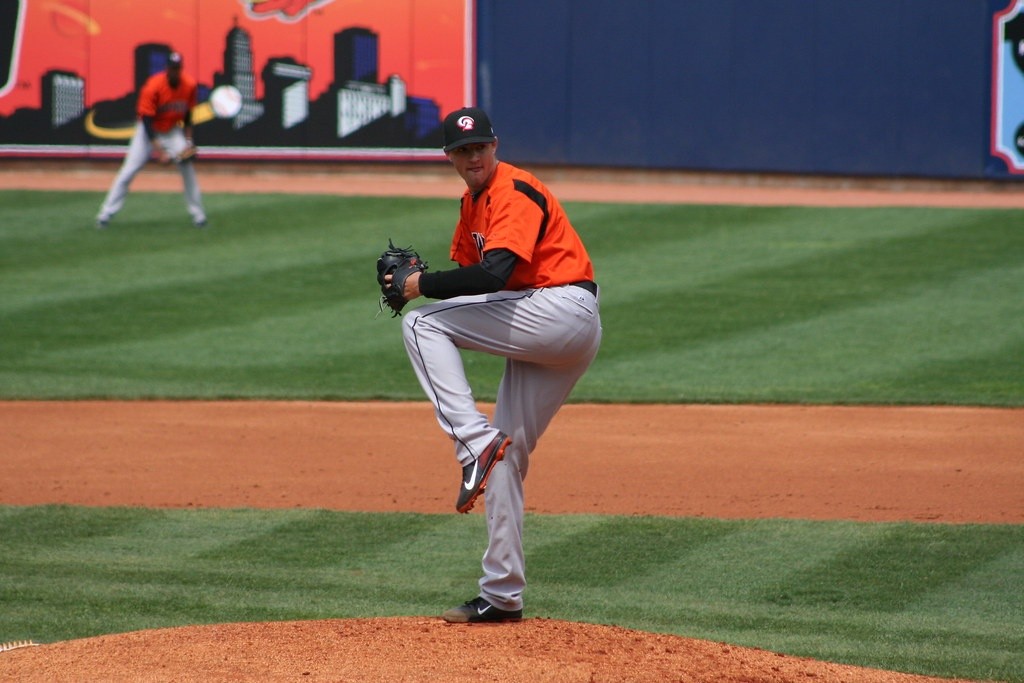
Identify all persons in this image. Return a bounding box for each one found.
[95,52,208,228]
[377,107,602,620]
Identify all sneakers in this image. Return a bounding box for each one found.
[455,431,512,514]
[444,598,523,624]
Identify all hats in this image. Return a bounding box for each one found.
[169,51,183,67]
[444,107,496,151]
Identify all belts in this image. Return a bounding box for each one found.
[569,277,597,297]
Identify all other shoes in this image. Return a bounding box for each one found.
[96,211,110,225]
[188,204,207,226]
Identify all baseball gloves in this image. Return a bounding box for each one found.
[177,145,197,163]
[373,237,430,322]
